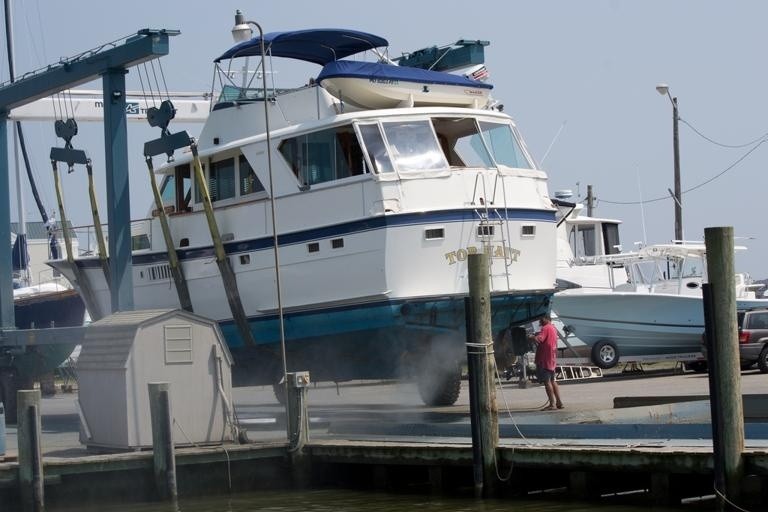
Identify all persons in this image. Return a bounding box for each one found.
[528,313,565,412]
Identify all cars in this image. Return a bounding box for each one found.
[703,307,768,374]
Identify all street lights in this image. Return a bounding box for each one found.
[656,84,682,245]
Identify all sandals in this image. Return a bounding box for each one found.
[541,402,564,411]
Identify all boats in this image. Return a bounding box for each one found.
[0,0,86,404]
[551,241,767,380]
[44,8,579,406]
